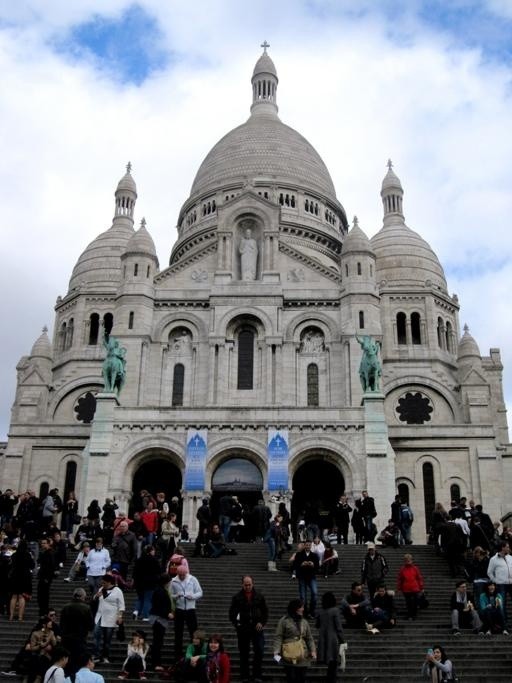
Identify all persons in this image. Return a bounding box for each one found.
[174,331,191,355]
[103,336,126,376]
[357,332,381,376]
[0,489,511,683]
[238,228,258,280]
[301,330,324,352]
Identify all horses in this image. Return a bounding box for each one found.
[357,335,380,392]
[102,335,126,398]
